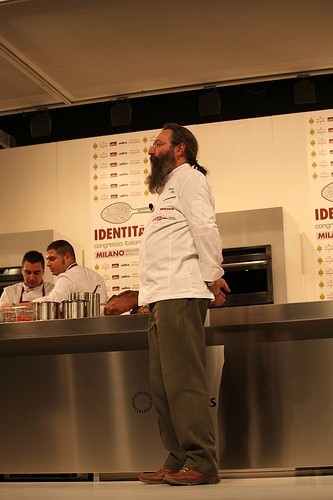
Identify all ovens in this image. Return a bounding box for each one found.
[221,244,273,306]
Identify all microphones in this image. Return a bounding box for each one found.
[149,203,153,210]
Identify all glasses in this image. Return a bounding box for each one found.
[153,140,172,147]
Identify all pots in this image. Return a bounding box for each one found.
[67,291,101,318]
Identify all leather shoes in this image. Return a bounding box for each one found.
[163,468,220,485]
[140,468,174,484]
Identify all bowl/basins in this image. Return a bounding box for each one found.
[32,301,62,320]
[62,300,91,319]
